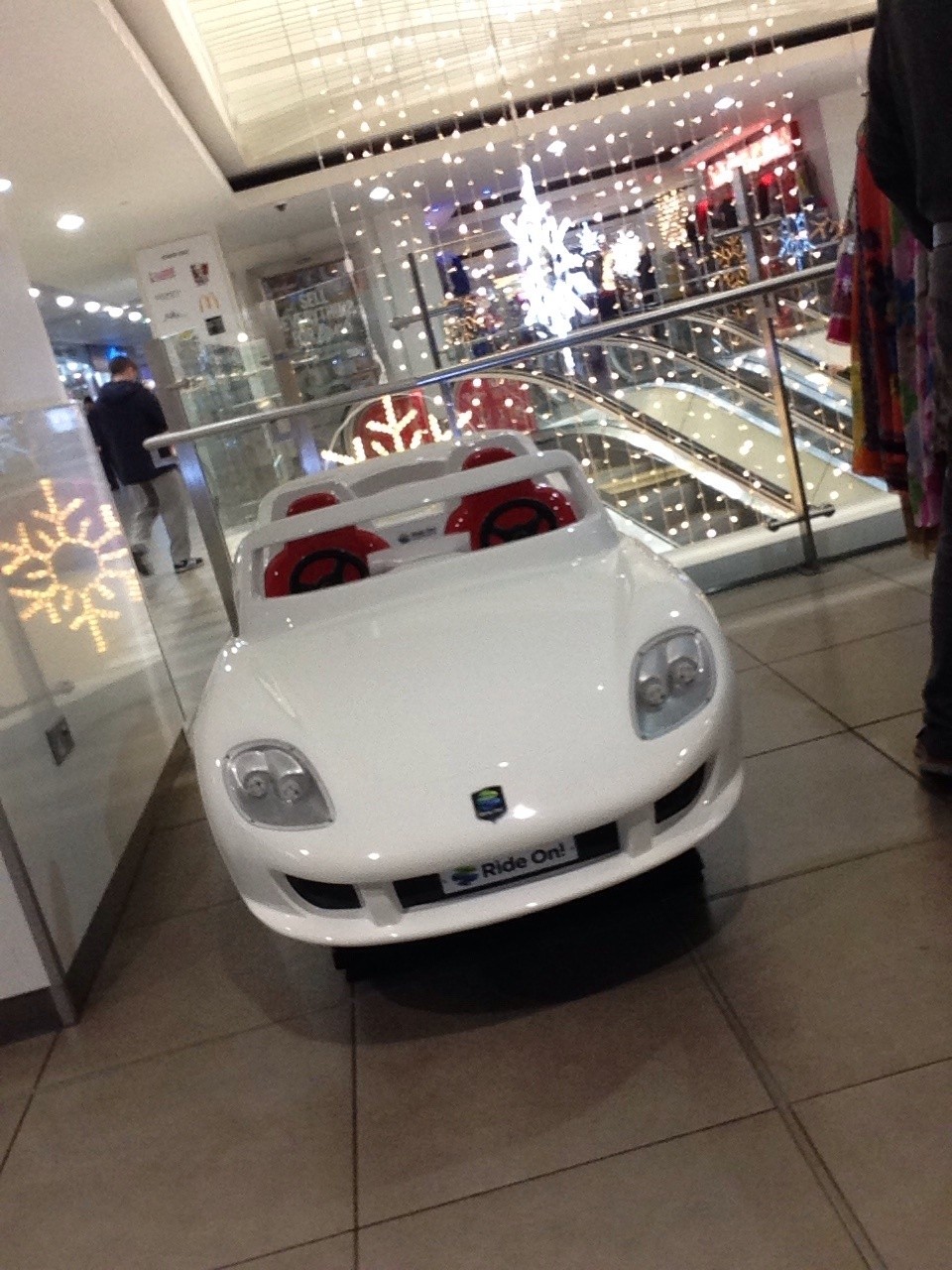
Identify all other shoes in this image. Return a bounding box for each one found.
[911,737,952,773]
[173,557,204,574]
[130,546,153,575]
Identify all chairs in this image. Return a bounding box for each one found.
[265,493,390,598]
[444,447,572,550]
[442,434,550,512]
[268,480,376,561]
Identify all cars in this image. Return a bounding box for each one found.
[186,421,746,948]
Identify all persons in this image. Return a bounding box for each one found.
[89,355,208,576]
[863,0,951,777]
[81,394,122,490]
